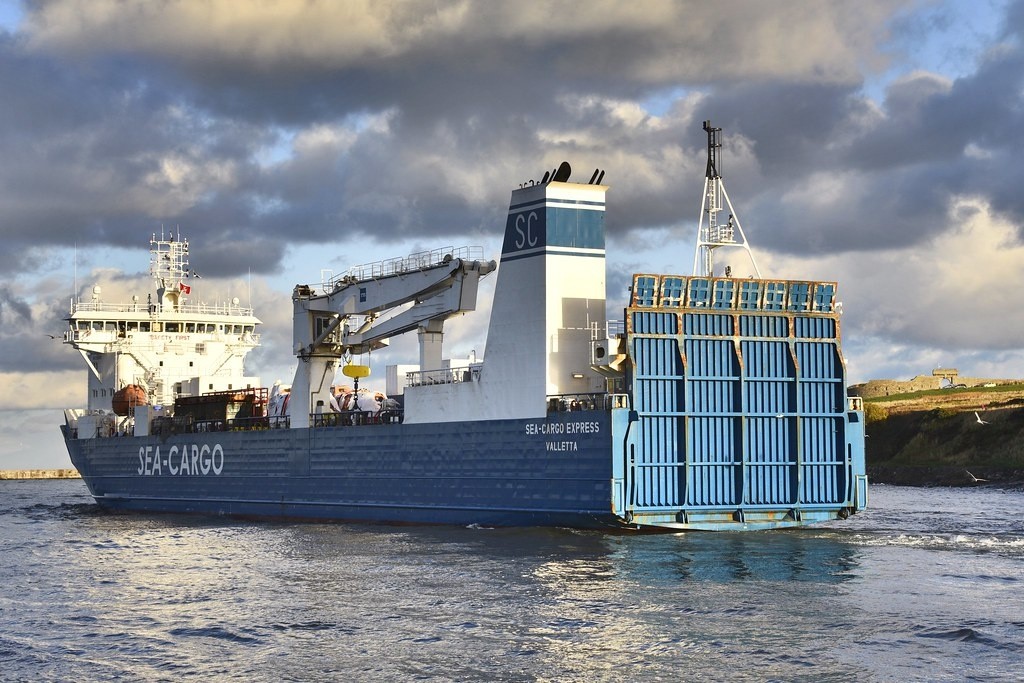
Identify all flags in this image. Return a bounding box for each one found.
[193,271,201,279]
[179,283,191,295]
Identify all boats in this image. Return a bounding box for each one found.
[111,384,147,417]
[58,119,870,534]
